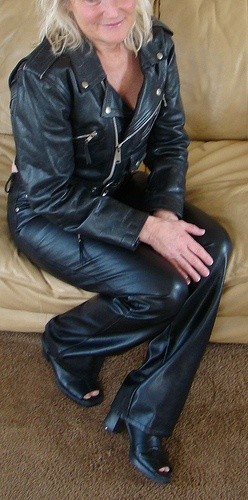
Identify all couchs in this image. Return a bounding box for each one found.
[0,0,247,345]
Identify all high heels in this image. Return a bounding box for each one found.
[101,387,175,483]
[40,325,107,407]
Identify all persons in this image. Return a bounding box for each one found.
[7,1,236,485]
